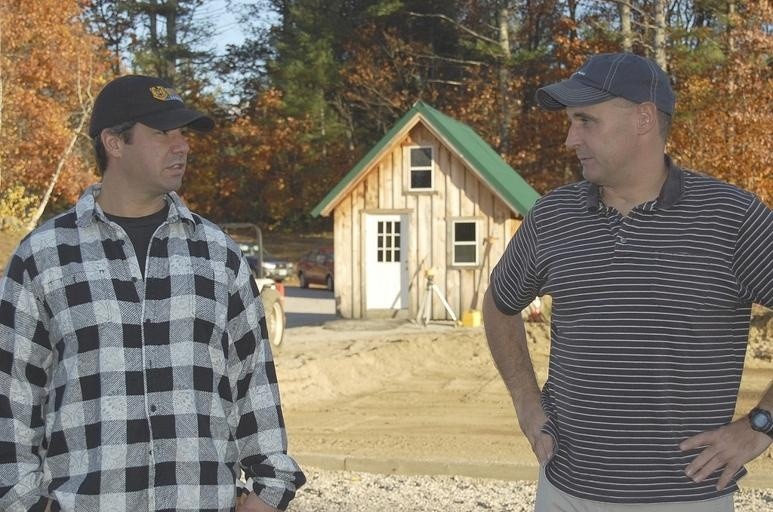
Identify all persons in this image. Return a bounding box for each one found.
[481,50,773,511]
[0,72,306,512]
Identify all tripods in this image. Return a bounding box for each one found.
[415,284,457,323]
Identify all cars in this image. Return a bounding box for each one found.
[235,242,334,291]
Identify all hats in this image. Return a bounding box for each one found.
[535,52,675,117]
[89,74,215,139]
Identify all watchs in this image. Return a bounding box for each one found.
[747,408,773,441]
